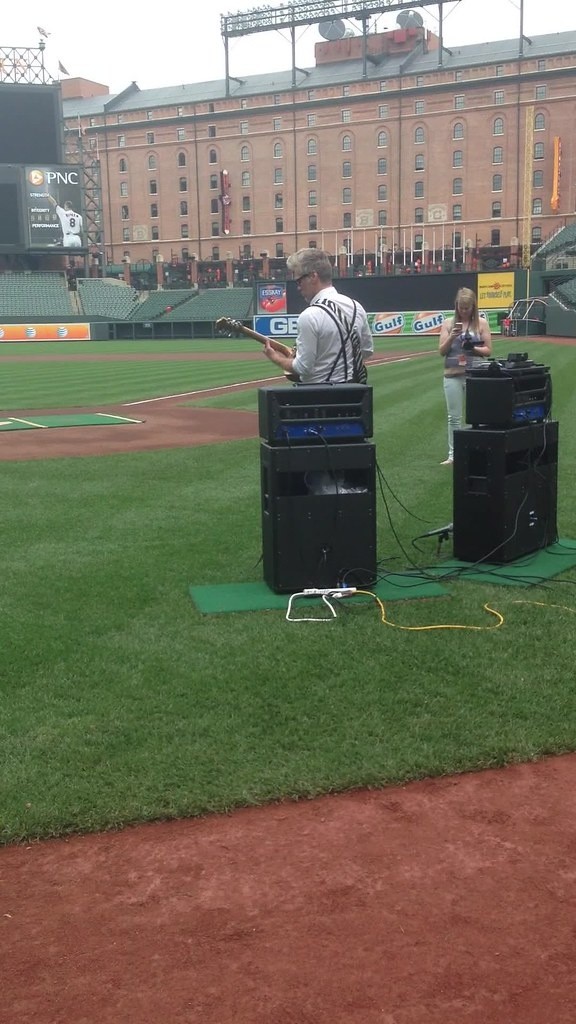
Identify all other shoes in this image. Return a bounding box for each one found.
[440,457,454,466]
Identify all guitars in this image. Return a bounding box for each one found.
[214,317,301,382]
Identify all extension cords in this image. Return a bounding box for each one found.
[304,587,357,597]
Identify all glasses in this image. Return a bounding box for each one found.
[291,271,319,286]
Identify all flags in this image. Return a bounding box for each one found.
[38,27,47,37]
[58,61,68,75]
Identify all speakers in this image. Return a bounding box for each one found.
[260,437,376,594]
[453,418,560,564]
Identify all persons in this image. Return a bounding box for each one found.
[438,287,493,464]
[45,184,83,248]
[264,249,374,385]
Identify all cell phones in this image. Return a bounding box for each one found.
[455,323,462,332]
